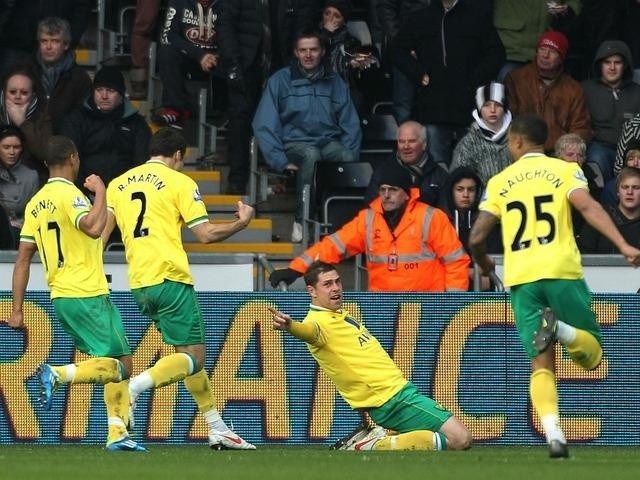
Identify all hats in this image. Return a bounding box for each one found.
[536,31,569,59]
[475,82,509,115]
[90,66,125,92]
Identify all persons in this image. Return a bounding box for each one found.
[1,0,640,296]
[467,115,639,460]
[266,259,474,453]
[5,135,149,455]
[101,128,259,453]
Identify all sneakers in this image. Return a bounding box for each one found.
[35,363,61,411]
[208,427,257,451]
[548,439,569,460]
[330,418,386,454]
[530,304,559,352]
[106,396,152,453]
[224,180,246,196]
[148,108,190,132]
[289,220,304,244]
